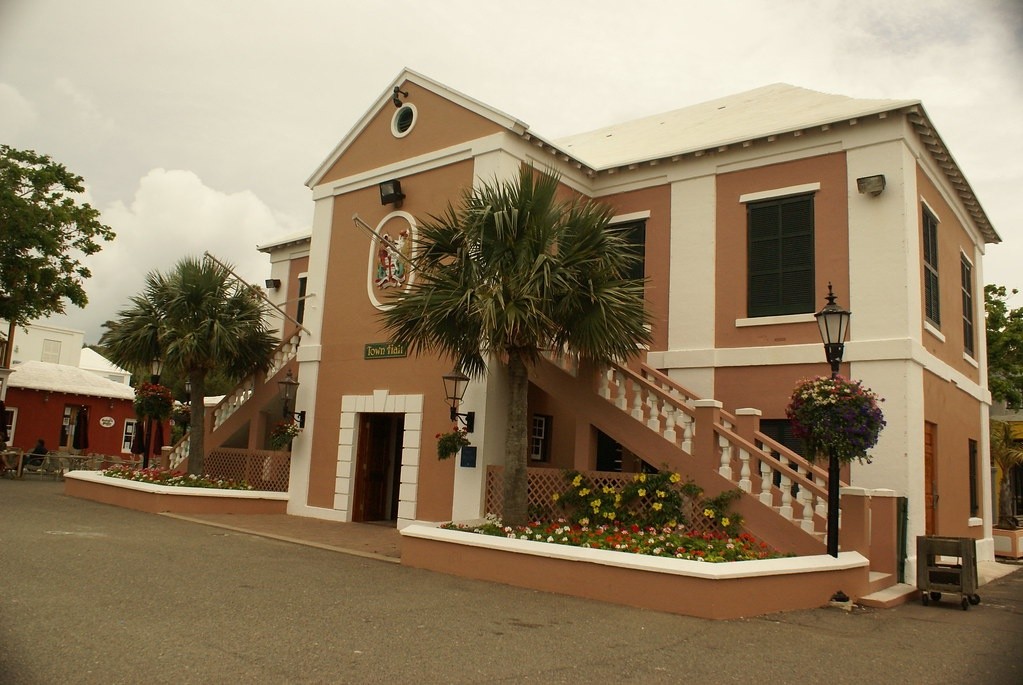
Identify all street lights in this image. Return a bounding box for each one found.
[812,282,852,557]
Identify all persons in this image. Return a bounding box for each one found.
[13,439,45,470]
[0,432,6,468]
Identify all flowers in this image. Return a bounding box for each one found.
[271,418,303,451]
[435,427,471,463]
[132,381,174,425]
[785,374,886,471]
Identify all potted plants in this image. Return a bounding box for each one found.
[989,417,1023,560]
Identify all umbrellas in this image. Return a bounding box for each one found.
[73,404,89,463]
[131,419,145,465]
[153,420,164,459]
[0,400,10,442]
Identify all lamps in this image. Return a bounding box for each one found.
[265,279,280,288]
[277,369,306,428]
[856,174,886,197]
[393,86,409,108]
[43,394,49,403]
[442,356,475,433]
[109,402,114,409]
[379,179,406,207]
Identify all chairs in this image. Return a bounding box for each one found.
[0,446,140,480]
